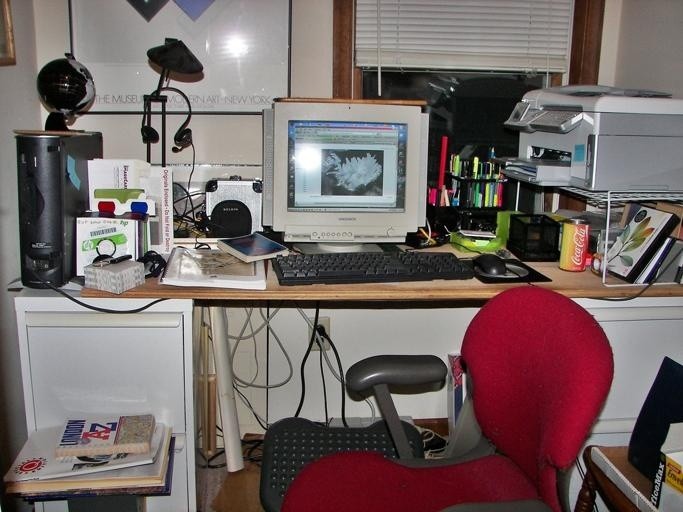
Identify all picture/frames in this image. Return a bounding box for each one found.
[69,0,292,114]
[0,0,17,66]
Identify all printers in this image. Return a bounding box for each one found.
[497,80,683,199]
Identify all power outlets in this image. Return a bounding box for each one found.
[308,318,330,351]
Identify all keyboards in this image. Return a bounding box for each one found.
[268,251,469,288]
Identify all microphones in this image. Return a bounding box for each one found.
[170,142,195,155]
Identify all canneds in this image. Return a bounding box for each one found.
[558,215,591,272]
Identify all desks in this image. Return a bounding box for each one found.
[14,219,683,512]
[574,445,683,511]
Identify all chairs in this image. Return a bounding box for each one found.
[282,287,614,511]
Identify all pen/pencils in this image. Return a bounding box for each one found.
[448,146,504,208]
[427,185,450,206]
[437,136,448,207]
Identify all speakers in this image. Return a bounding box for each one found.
[204,176,263,243]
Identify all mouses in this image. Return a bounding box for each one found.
[473,250,509,277]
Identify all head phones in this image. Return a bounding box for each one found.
[140,87,194,147]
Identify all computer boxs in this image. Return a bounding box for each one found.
[16,132,104,288]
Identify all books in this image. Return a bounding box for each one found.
[606,201,683,284]
[216,231,290,264]
[3,413,176,500]
[75,215,152,278]
[158,246,267,291]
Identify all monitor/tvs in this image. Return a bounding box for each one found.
[263,97,430,252]
